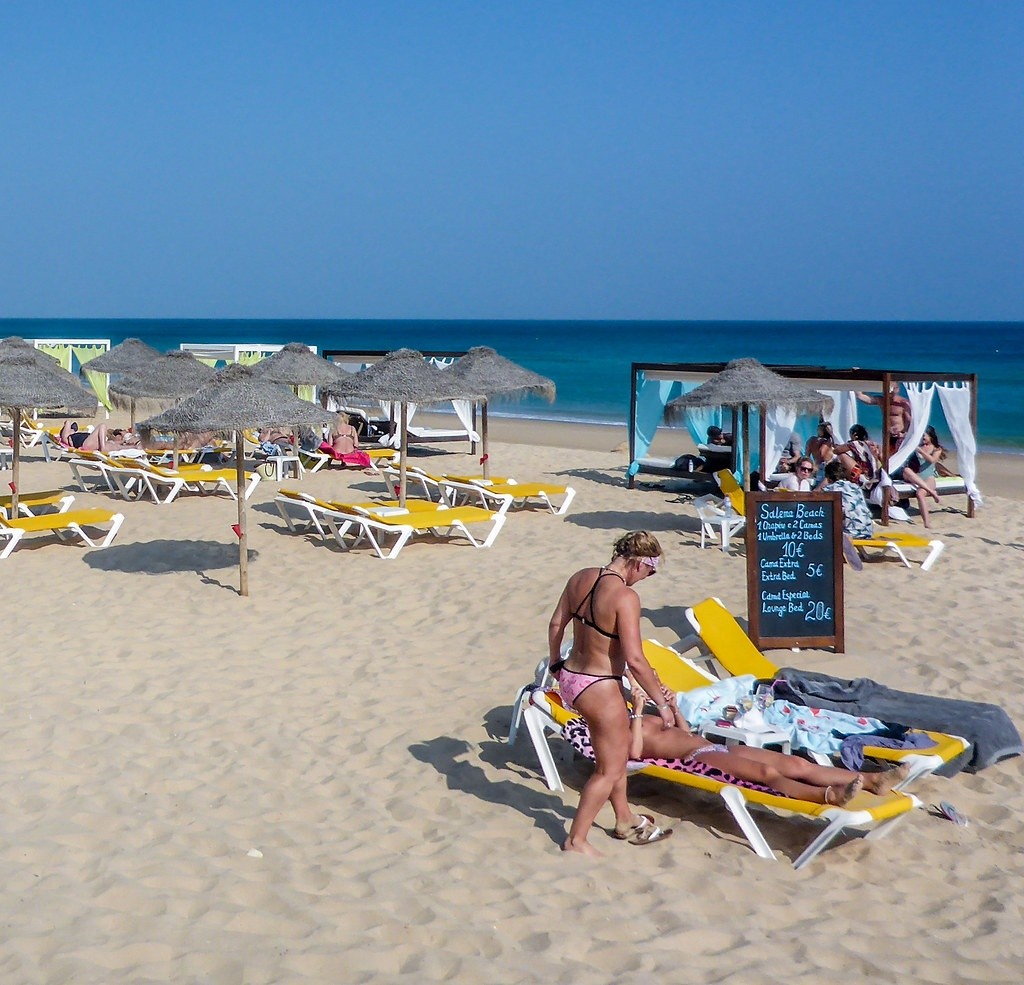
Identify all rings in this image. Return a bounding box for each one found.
[667,722,671,726]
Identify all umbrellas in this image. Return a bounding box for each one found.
[0,336,215,518]
[318,346,555,507]
[663,357,834,492]
[135,362,342,595]
[250,341,352,478]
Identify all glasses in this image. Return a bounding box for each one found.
[647,554,657,577]
[798,466,814,472]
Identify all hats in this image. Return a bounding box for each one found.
[706,425,723,445]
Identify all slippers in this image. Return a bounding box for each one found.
[942,506,967,516]
[934,799,967,827]
[628,824,673,846]
[612,813,654,840]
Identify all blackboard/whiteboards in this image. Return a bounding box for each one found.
[744,490,844,648]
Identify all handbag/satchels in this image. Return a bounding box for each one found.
[260,442,278,456]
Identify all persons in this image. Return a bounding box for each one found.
[622,688,911,808]
[691,379,958,539]
[547,531,665,860]
[62,412,359,456]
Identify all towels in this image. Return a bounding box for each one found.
[318,442,370,467]
[753,666,1023,778]
[761,697,888,756]
[840,729,938,771]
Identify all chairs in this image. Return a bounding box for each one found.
[0,406,577,560]
[694,470,945,571]
[508,597,1024,870]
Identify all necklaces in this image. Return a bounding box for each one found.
[602,566,627,586]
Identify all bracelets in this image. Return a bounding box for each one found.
[656,703,669,710]
[630,714,642,718]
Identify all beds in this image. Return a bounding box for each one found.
[628,362,984,526]
[323,350,477,455]
